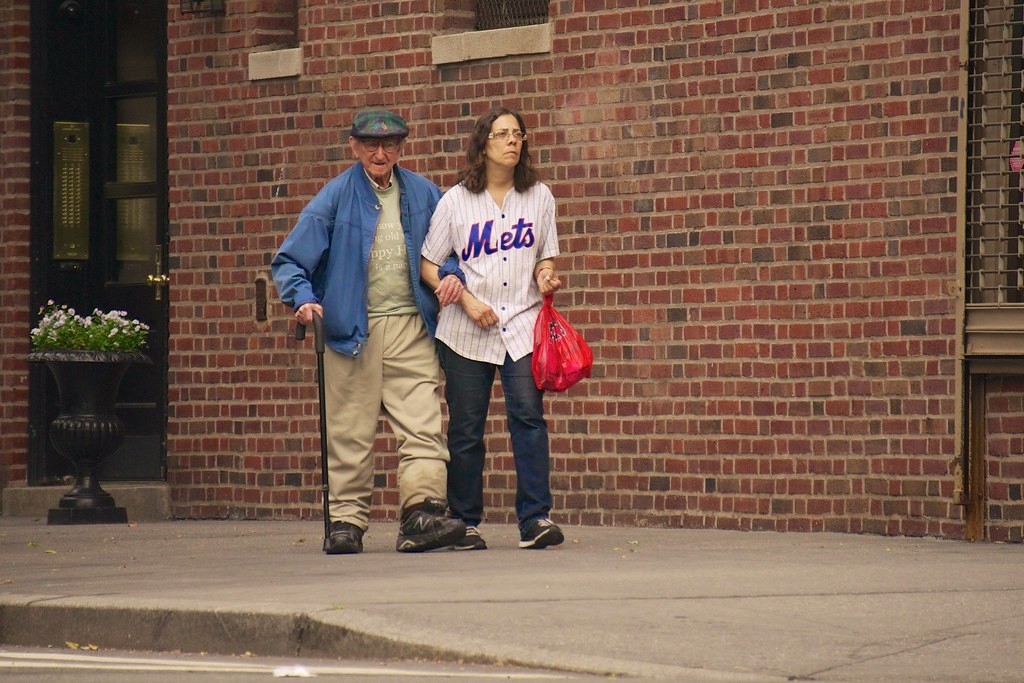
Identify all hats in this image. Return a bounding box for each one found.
[351,109,409,138]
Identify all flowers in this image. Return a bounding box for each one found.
[31,298,150,352]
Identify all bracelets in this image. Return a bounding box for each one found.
[537,266,551,276]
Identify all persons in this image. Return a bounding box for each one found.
[422,109,564,550]
[268,109,466,554]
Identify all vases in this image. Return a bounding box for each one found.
[26,352,155,523]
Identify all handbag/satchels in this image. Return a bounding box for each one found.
[532,267,593,392]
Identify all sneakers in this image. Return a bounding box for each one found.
[519,518,564,549]
[324,520,363,554]
[396,497,465,551]
[453,525,487,550]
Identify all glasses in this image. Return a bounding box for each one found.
[487,131,527,141]
[362,139,399,153]
